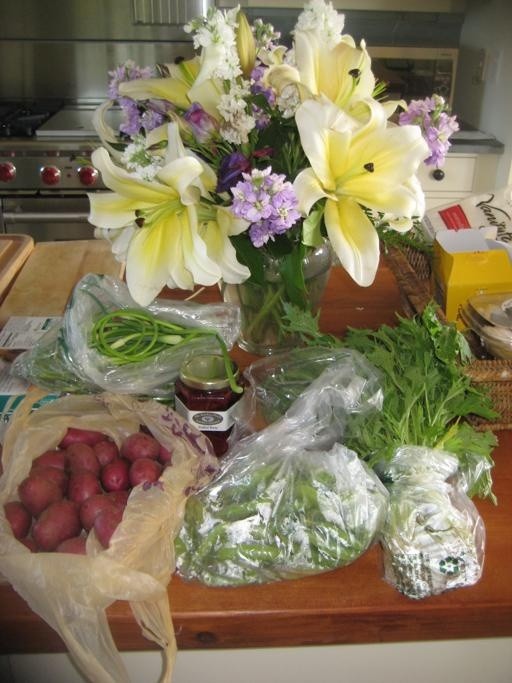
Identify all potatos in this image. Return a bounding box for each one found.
[5,429,172,554]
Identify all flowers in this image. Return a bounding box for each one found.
[85,0,460,347]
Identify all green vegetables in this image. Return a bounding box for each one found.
[258,298,502,599]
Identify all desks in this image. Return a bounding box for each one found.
[0,234,511,655]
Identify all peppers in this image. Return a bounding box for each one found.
[174,459,378,588]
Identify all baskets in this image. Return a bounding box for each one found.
[377,207,512,433]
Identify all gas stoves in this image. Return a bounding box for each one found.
[0,98,141,191]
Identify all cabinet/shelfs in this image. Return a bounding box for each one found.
[413,153,501,215]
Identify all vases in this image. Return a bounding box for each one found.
[215,237,334,357]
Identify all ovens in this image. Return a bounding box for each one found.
[0,188,123,243]
